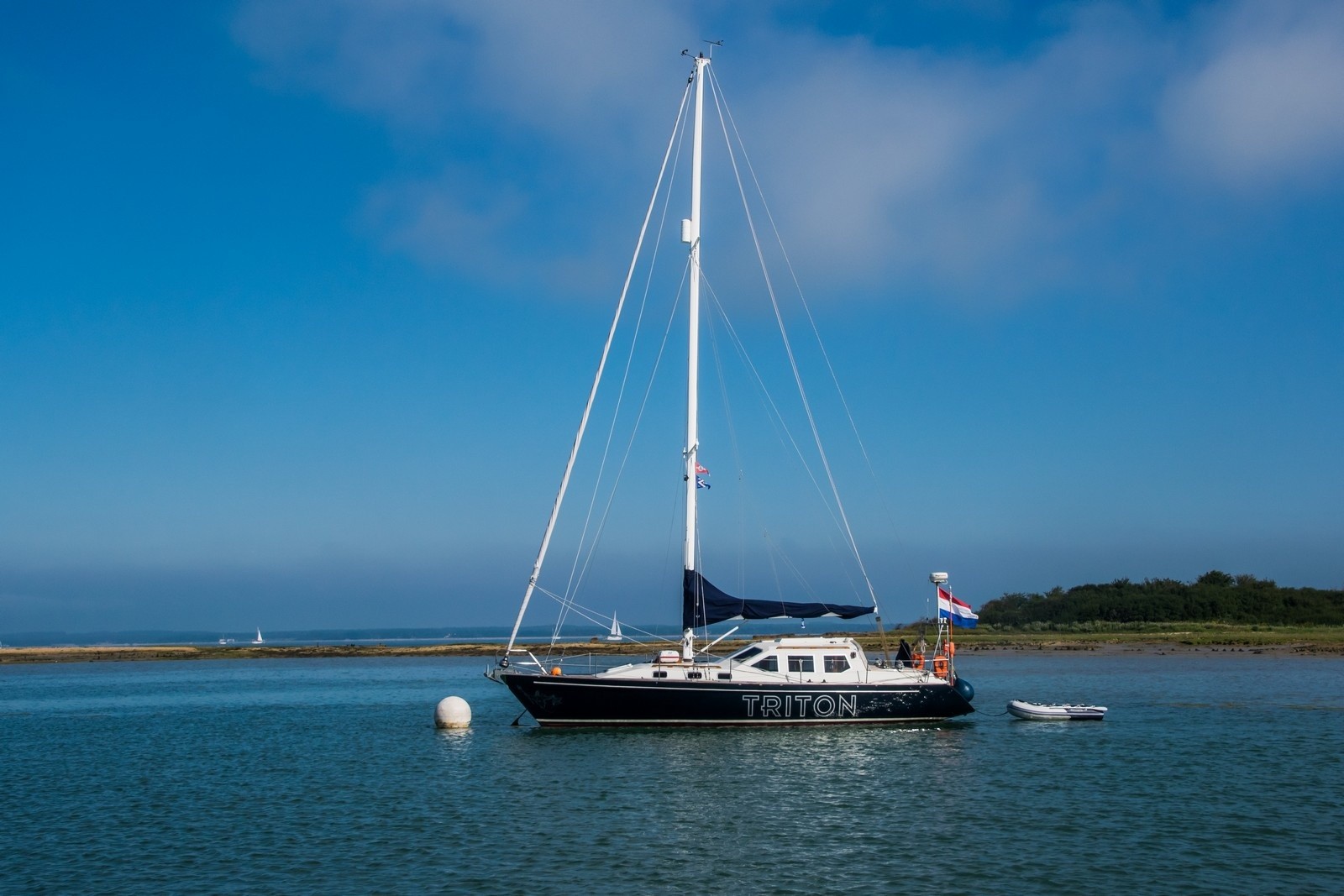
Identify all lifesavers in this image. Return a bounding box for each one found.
[911,654,925,669]
[934,655,948,677]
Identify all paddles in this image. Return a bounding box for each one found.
[1049,706,1108,711]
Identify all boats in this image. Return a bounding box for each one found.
[1006,699,1108,721]
[219,634,234,644]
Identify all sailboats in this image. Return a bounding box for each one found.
[606,608,623,641]
[484,38,978,726]
[251,627,266,644]
